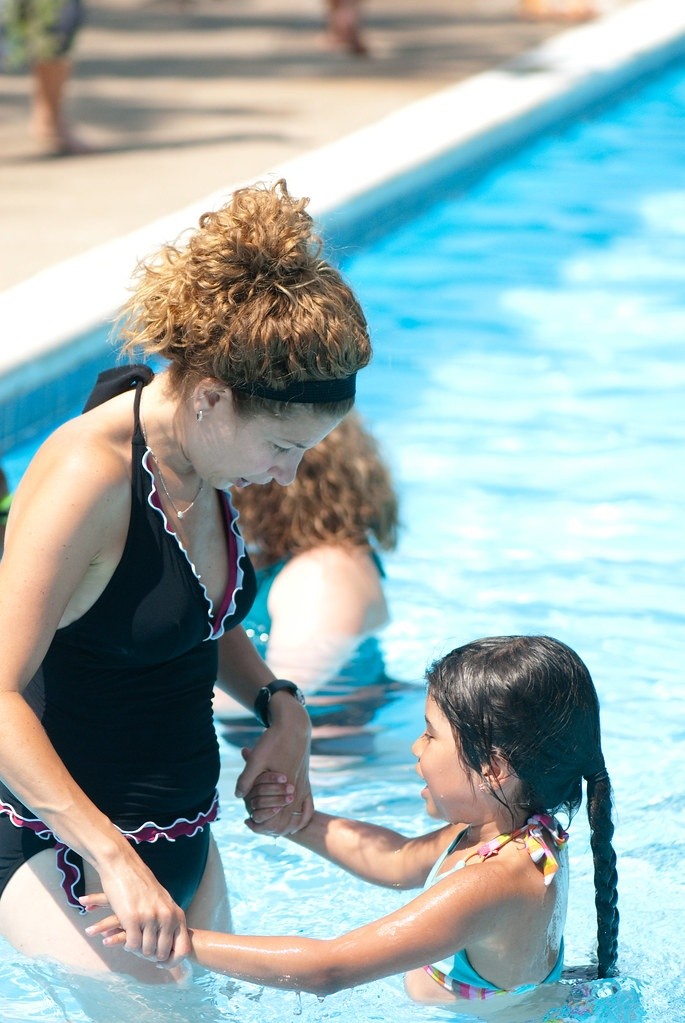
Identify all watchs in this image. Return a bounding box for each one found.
[252,679,305,728]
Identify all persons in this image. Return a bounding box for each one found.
[75,634,622,1004]
[230,405,399,687]
[0,181,373,987]
